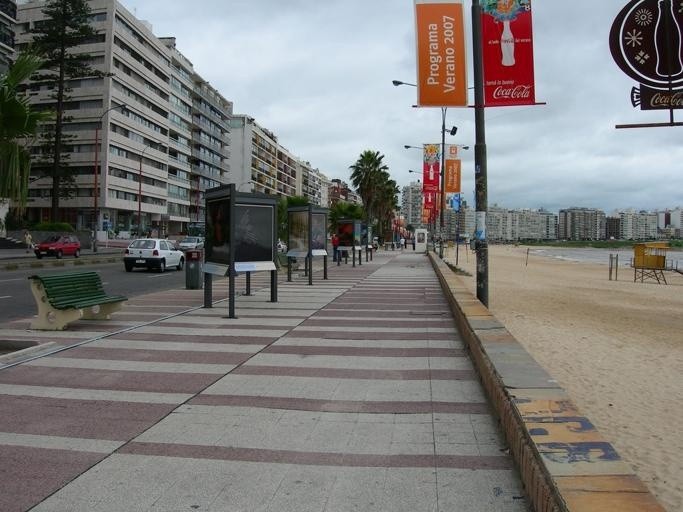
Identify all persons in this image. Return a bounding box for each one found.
[331,234,340,262]
[22,232,32,253]
[399,236,405,250]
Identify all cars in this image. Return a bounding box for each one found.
[32,231,82,259]
[276,240,288,253]
[122,236,205,272]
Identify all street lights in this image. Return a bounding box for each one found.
[236,182,253,192]
[91,103,127,254]
[136,141,166,236]
[389,80,476,260]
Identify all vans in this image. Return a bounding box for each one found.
[372,236,379,249]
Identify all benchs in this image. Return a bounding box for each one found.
[25,271,128,330]
[275,253,299,275]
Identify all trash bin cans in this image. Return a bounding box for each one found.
[186,248,204,289]
